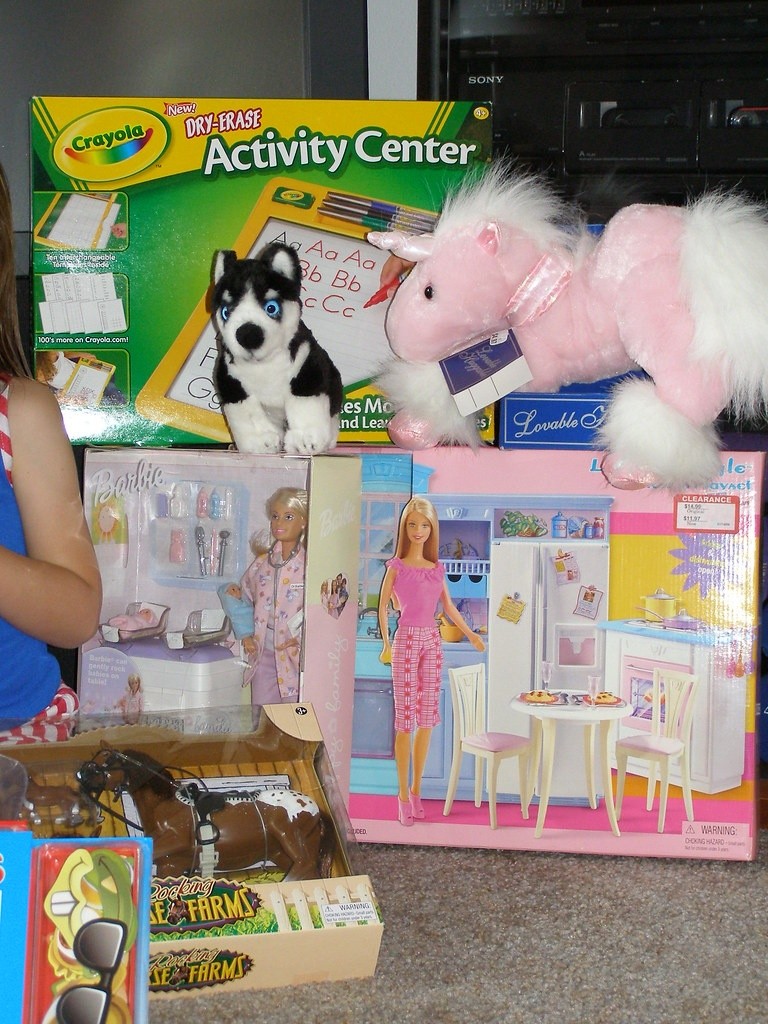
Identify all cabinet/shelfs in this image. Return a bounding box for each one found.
[350,488,615,807]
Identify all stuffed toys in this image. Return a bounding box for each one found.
[366,156,768,490]
[211,243,343,455]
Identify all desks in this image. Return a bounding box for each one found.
[512,689,634,836]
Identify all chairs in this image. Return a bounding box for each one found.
[445,662,531,829]
[616,668,703,833]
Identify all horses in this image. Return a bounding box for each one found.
[79,751,339,882]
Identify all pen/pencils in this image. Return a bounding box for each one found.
[317,191,436,237]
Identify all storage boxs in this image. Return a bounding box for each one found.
[28,95,499,446]
[0,702,384,1000]
[322,443,768,861]
[497,223,649,450]
[78,445,365,812]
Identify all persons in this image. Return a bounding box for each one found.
[217,486,305,730]
[0,162,103,743]
